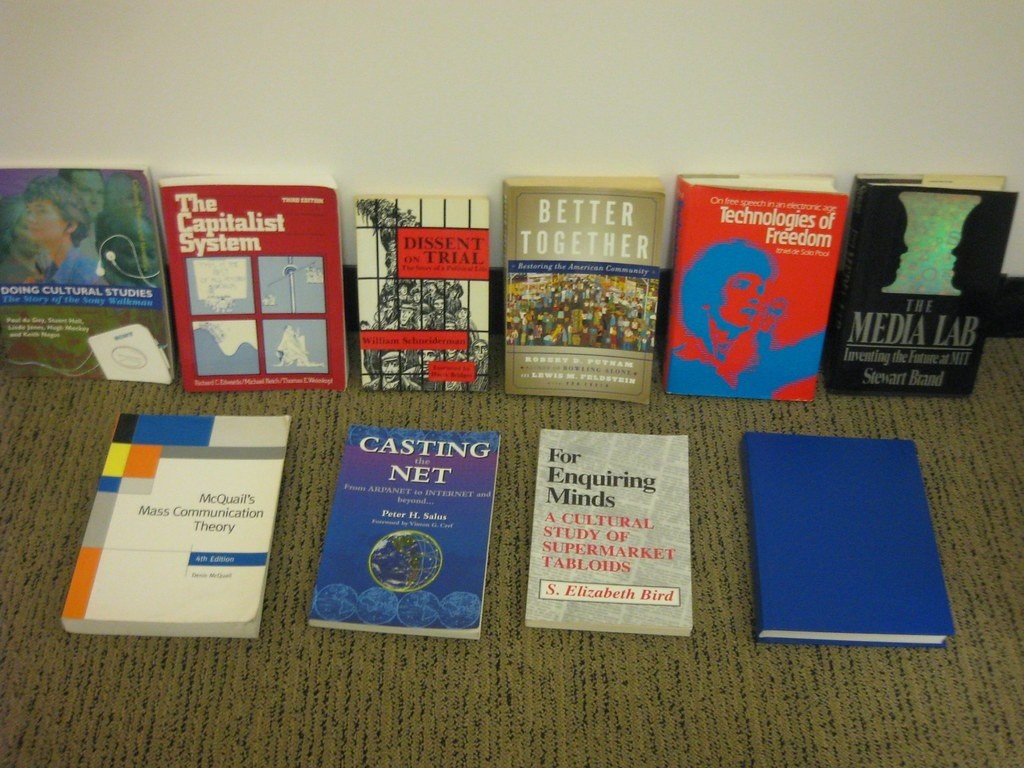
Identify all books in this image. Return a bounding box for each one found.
[0,168,174,386]
[739,431,955,651]
[520,425,697,640]
[158,166,1018,405]
[60,413,295,640]
[306,420,496,643]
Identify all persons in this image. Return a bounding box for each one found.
[2,171,150,288]
